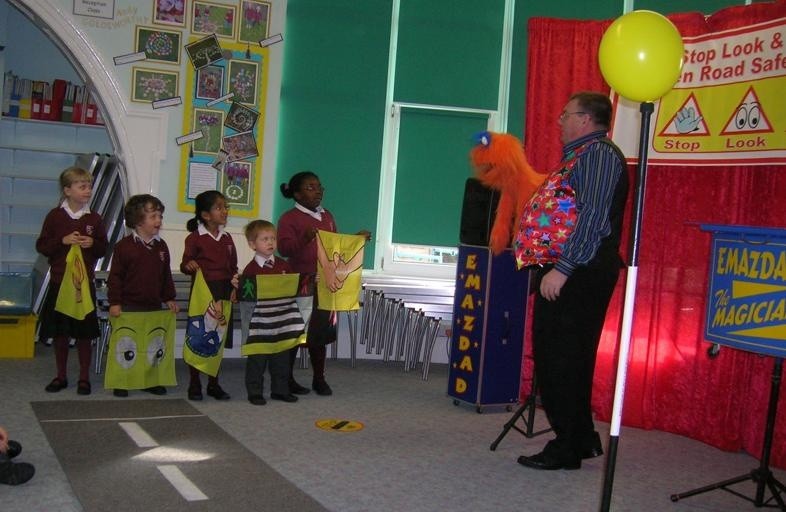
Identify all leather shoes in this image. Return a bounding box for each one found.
[0,440,21,461]
[581,432,603,457]
[46,377,332,405]
[0,462,35,484]
[518,440,583,470]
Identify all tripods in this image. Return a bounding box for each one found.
[670,357,786,512]
[489,368,554,450]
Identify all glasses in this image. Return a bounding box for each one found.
[558,112,586,119]
[211,205,230,211]
[302,186,325,191]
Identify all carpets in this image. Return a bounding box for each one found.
[29,398,332,511]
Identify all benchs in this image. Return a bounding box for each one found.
[93,270,308,375]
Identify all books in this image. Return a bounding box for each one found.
[2,73,105,126]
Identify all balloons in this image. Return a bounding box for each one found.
[598,9,685,106]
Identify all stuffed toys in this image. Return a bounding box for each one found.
[470,130,550,260]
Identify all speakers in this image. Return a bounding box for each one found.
[458,176,515,247]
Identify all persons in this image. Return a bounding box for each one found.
[0,422,36,489]
[198,113,219,127]
[231,218,301,406]
[101,190,183,400]
[276,171,372,399]
[244,2,263,31]
[176,187,240,405]
[194,4,233,34]
[31,166,109,399]
[226,161,250,188]
[512,89,632,473]
